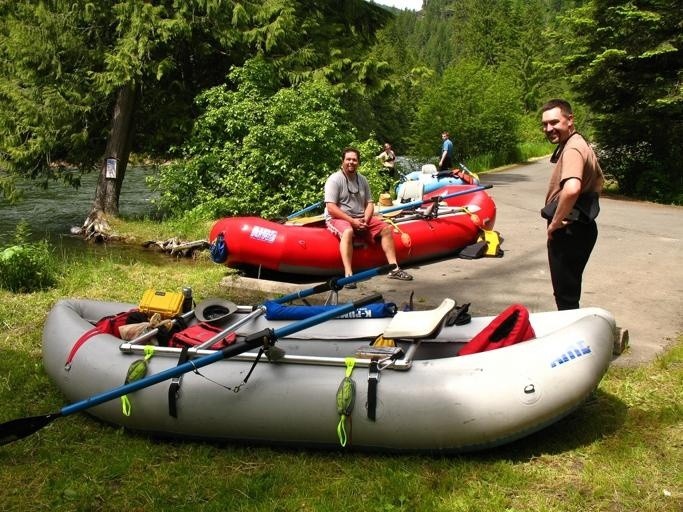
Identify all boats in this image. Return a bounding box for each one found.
[40,294,631,452]
[394,167,479,198]
[208,180,498,277]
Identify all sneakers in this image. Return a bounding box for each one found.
[345,281,355,288]
[388,271,412,280]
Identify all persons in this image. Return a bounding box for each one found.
[321,146,413,289]
[376,142,396,193]
[437,130,455,172]
[538,98,604,310]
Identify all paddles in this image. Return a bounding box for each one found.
[0,294,382,448]
[297,184,493,224]
